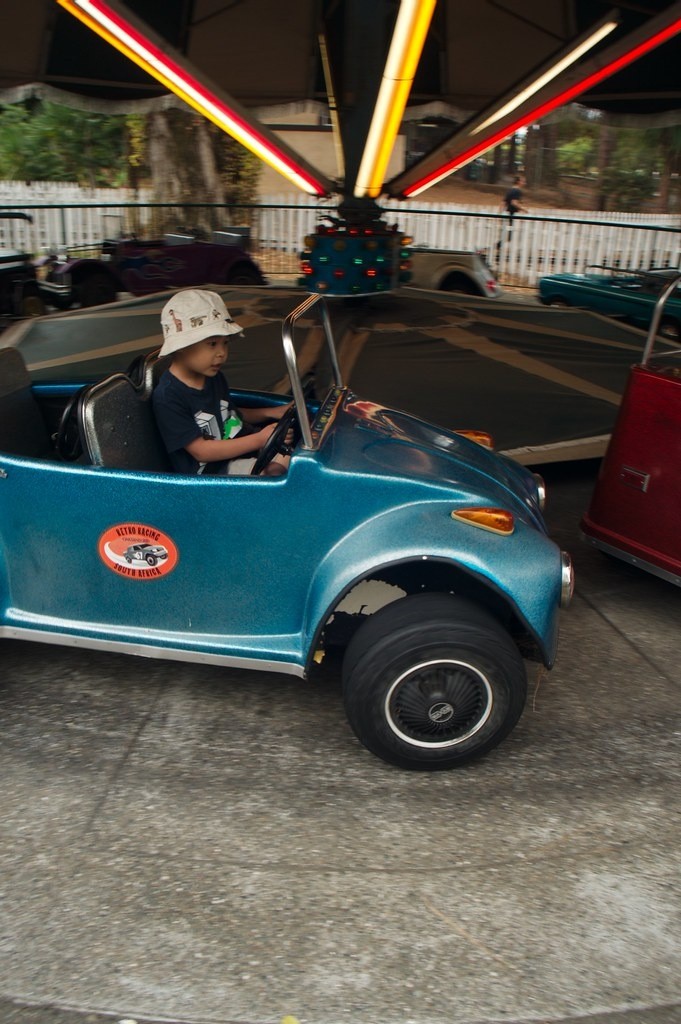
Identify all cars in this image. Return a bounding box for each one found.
[538,264,681,341]
[53,223,268,307]
[0,289,577,774]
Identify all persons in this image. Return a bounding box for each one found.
[494,175,530,266]
[141,289,297,478]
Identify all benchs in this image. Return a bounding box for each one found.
[0,347,177,474]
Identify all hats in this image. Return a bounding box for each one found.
[158,288,245,358]
